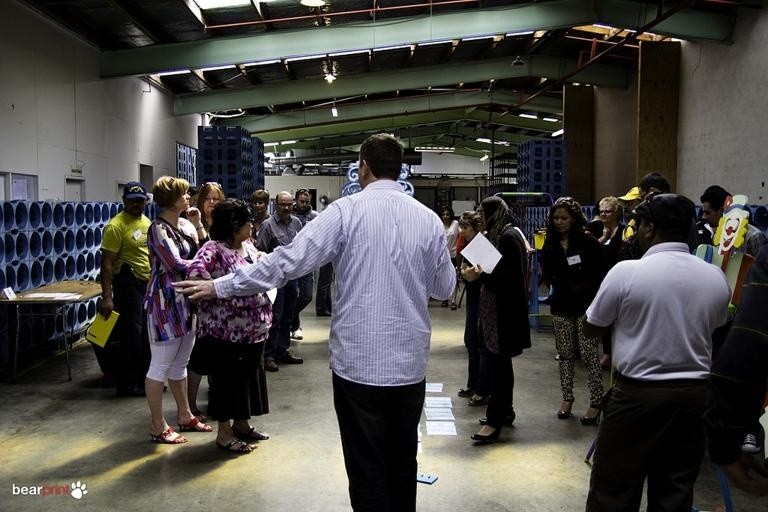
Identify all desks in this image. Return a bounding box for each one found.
[0,281,112,382]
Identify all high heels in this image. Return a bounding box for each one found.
[558,398,575,419]
[582,402,603,424]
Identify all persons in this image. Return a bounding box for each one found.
[85,133,768,512]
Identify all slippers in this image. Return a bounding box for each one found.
[177,416,212,432]
[149,427,187,444]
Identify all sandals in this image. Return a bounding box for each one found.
[191,410,207,423]
[216,434,252,453]
[233,426,269,440]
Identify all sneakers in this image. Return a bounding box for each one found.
[292,327,303,340]
[441,301,448,307]
[117,384,145,397]
[742,434,761,452]
[451,302,457,310]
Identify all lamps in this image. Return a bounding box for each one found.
[323,57,336,83]
[332,106,338,117]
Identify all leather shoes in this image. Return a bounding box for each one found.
[458,385,515,441]
[264,359,278,371]
[282,352,303,363]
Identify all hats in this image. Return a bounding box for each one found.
[123,182,147,200]
[617,186,640,201]
[632,192,695,226]
